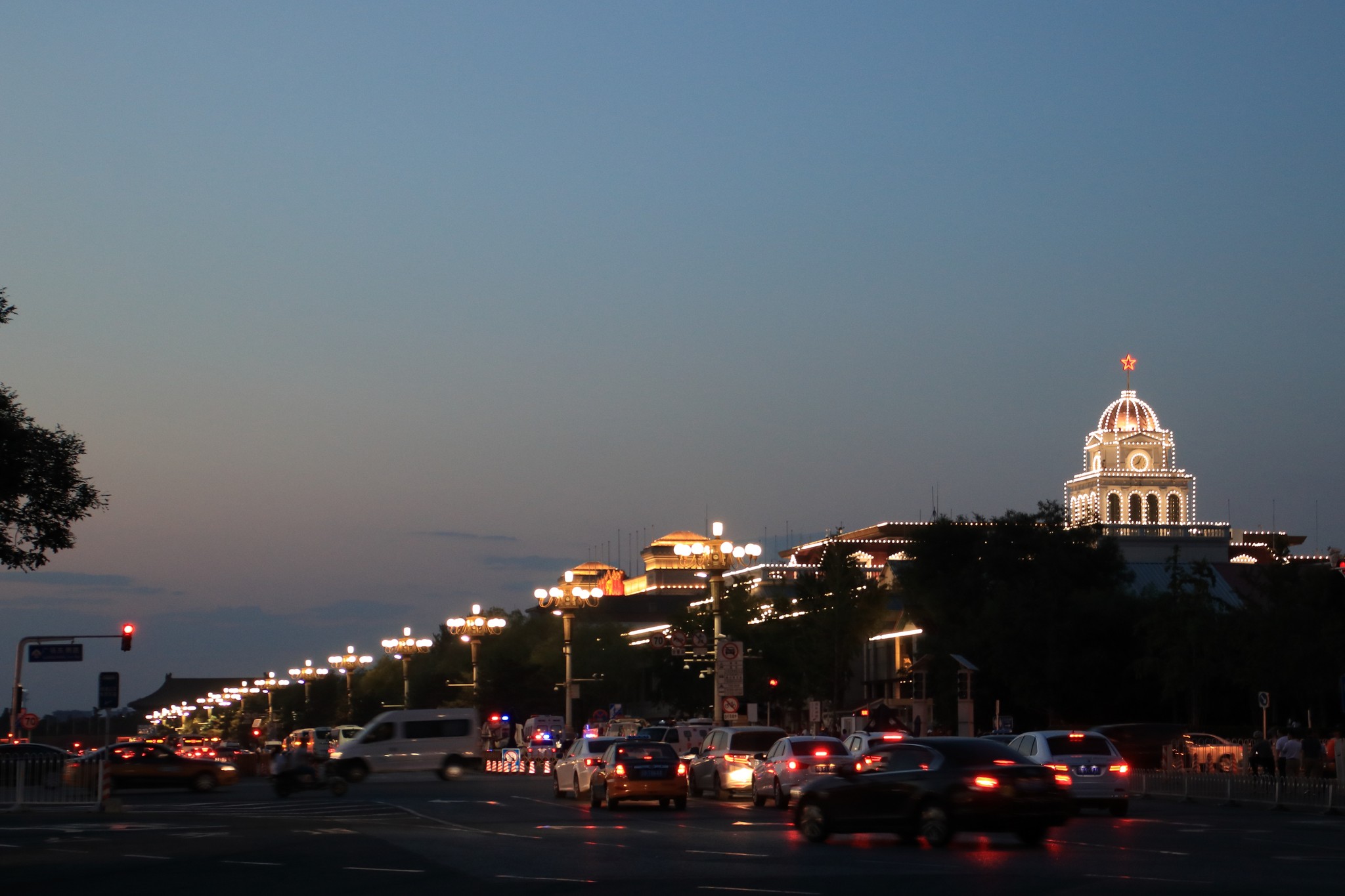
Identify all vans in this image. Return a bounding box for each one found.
[330,708,482,782]
[480,713,566,747]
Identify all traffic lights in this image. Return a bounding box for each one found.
[861,709,870,716]
[121,624,133,651]
[751,730,1133,851]
[769,678,779,689]
[253,729,260,737]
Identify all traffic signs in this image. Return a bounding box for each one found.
[993,716,1013,730]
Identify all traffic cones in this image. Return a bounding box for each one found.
[485,760,552,776]
[1161,745,1238,774]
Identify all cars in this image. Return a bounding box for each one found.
[264,726,363,757]
[1090,724,1254,773]
[551,717,716,810]
[0,737,256,793]
[689,727,790,802]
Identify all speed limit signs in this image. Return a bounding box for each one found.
[649,632,667,650]
[21,714,38,730]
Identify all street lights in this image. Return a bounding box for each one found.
[327,644,375,725]
[288,657,329,726]
[144,680,260,742]
[253,670,290,726]
[674,521,762,729]
[380,625,433,711]
[444,600,506,709]
[530,564,605,744]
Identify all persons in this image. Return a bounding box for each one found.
[255,731,320,791]
[795,708,849,742]
[482,717,499,749]
[661,712,679,727]
[1248,727,1341,790]
[640,717,652,729]
[862,714,986,737]
